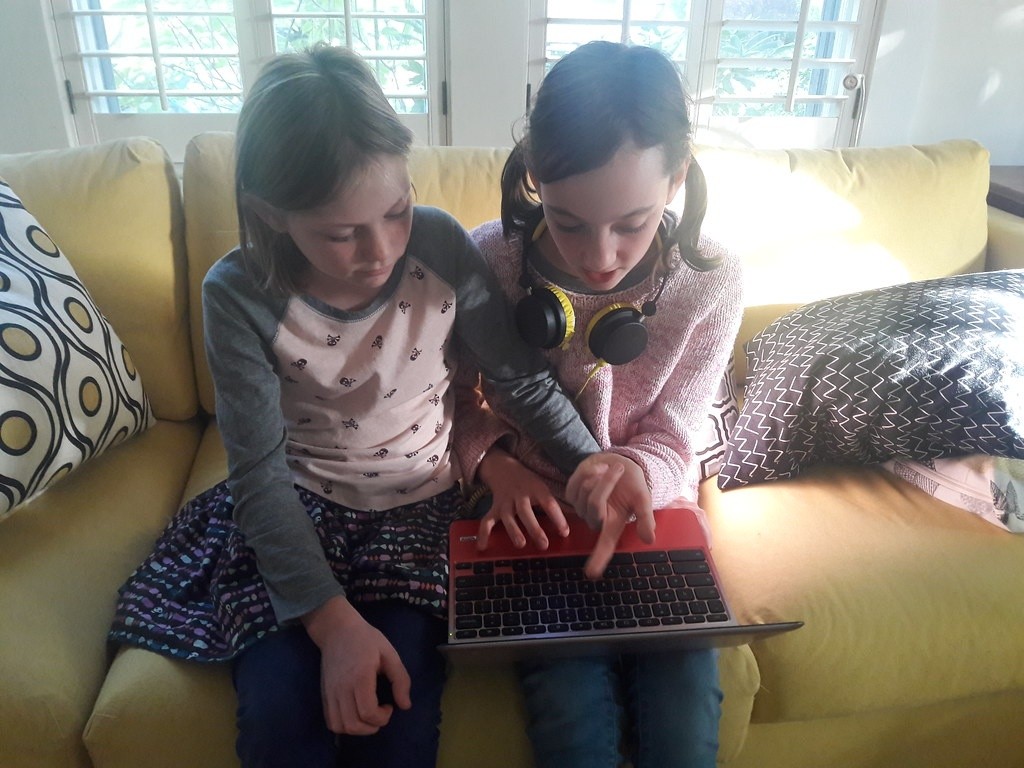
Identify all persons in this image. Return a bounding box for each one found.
[104,44,604,768]
[447,40,742,768]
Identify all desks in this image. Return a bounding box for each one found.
[984,165,1024,272]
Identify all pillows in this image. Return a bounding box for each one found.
[716,269,1024,492]
[735,375,1024,535]
[0,178,158,517]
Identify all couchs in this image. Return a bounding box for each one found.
[0,131,1024,768]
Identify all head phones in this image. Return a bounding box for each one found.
[514,203,679,364]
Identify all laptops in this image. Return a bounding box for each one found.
[437,509,802,672]
